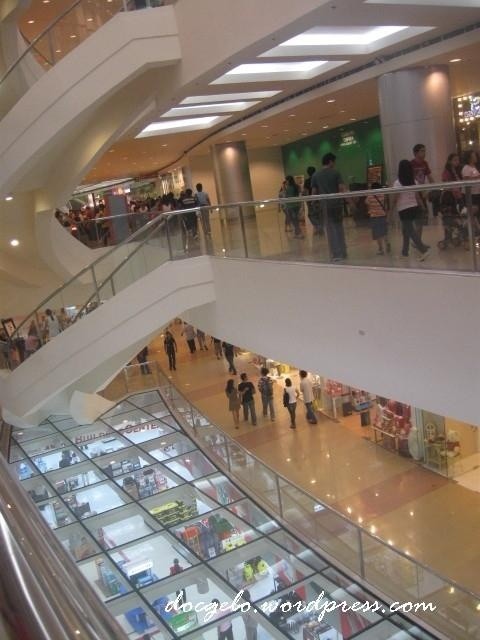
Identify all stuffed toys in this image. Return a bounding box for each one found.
[440,430,460,458]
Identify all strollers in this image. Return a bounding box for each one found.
[432,192,477,253]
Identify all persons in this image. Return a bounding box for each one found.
[181,322,237,375]
[0,302,102,370]
[54,182,213,240]
[278,143,480,262]
[163,332,177,371]
[170,559,186,610]
[137,347,152,375]
[59,450,90,488]
[240,590,258,640]
[258,368,275,422]
[283,378,299,429]
[212,600,234,640]
[299,371,317,424]
[225,379,249,429]
[238,373,257,425]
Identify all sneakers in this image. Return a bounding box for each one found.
[330,242,432,264]
[230,415,319,431]
